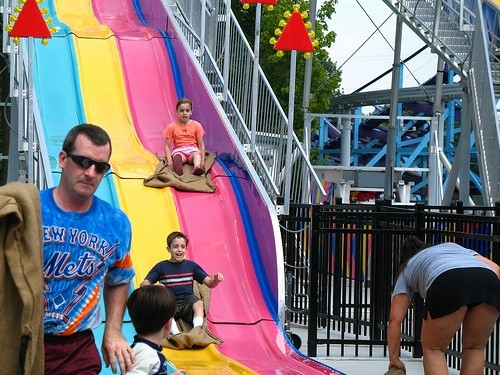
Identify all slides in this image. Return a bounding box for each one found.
[16,0,347,375]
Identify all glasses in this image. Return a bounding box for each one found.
[67,153,110,174]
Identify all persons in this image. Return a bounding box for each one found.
[140,231,224,349]
[161,99,206,175]
[387,236,500,375]
[0,124,137,375]
[122,284,186,375]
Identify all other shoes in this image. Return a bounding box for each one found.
[192,166,204,176]
[174,153,183,176]
